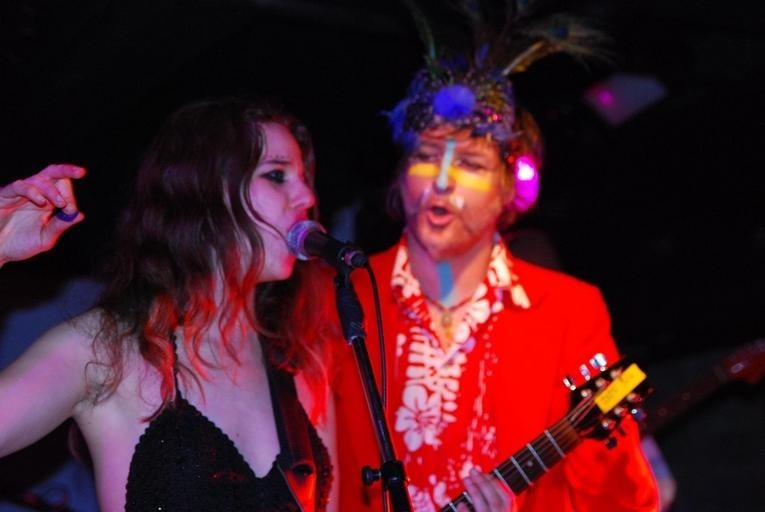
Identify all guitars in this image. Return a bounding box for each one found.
[648,338,765,430]
[432,353,653,512]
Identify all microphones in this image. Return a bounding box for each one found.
[286,220,368,269]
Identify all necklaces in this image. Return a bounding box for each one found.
[423,294,475,329]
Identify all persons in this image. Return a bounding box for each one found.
[0,102,518,512]
[323,60,661,512]
